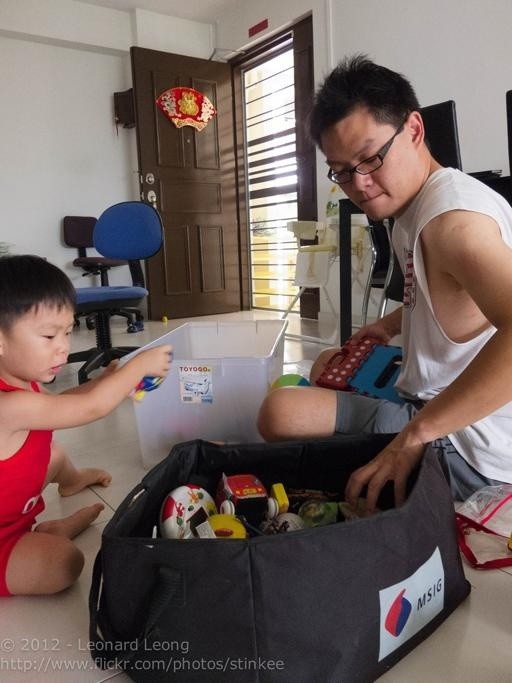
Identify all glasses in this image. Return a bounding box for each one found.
[328,110,413,184]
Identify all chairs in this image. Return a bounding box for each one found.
[64,215,145,333]
[44,202,164,386]
[361,214,406,327]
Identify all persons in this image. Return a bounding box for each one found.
[1,256,171,598]
[256,52,511,516]
[158,472,387,540]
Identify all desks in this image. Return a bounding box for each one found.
[339,175,511,347]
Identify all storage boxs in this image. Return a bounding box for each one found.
[121,317,290,467]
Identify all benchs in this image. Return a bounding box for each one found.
[279,203,380,348]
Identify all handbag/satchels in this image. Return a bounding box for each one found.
[88,432,471,683]
